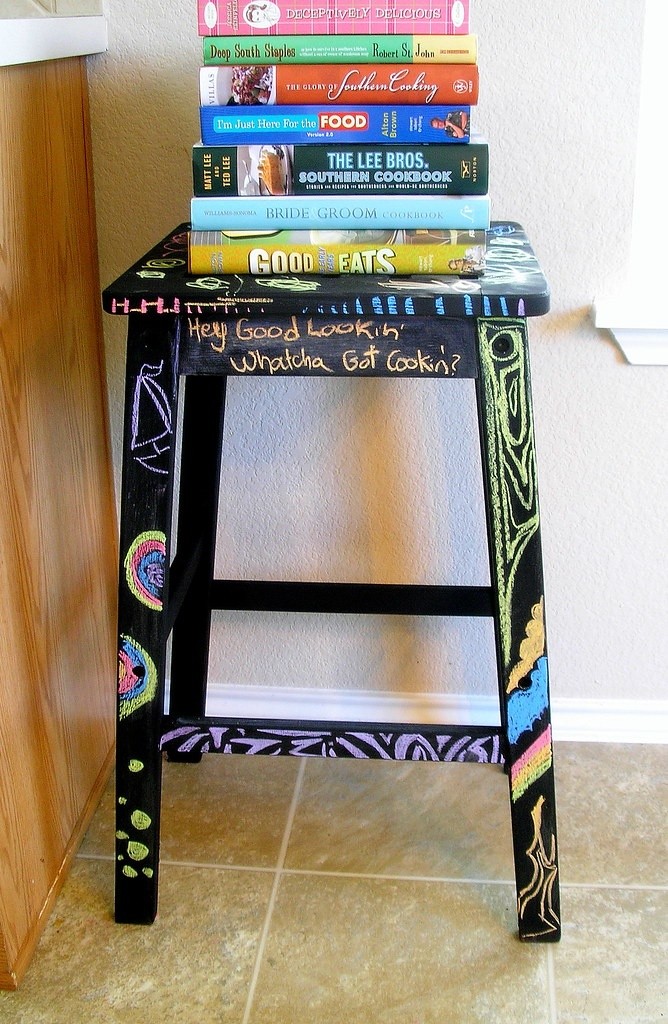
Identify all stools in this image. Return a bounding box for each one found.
[101,222,562,943]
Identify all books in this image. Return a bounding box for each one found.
[188,0,493,276]
